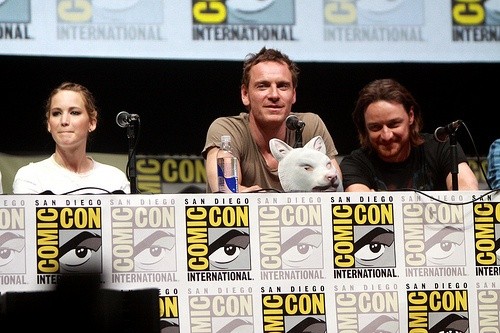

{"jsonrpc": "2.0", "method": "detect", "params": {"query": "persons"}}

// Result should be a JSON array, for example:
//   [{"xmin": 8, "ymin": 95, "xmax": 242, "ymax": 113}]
[
  {"xmin": 13, "ymin": 82, "xmax": 131, "ymax": 195},
  {"xmin": 338, "ymin": 79, "xmax": 478, "ymax": 192},
  {"xmin": 487, "ymin": 139, "xmax": 500, "ymax": 191},
  {"xmin": 201, "ymin": 45, "xmax": 342, "ymax": 194}
]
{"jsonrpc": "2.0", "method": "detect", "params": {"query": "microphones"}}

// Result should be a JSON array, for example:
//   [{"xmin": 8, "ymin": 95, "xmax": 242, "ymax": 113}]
[
  {"xmin": 115, "ymin": 111, "xmax": 139, "ymax": 129},
  {"xmin": 433, "ymin": 119, "xmax": 461, "ymax": 143},
  {"xmin": 284, "ymin": 114, "xmax": 304, "ymax": 130}
]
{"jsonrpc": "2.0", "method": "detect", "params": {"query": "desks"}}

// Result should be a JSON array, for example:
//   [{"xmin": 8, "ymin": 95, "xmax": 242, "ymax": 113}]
[{"xmin": 0, "ymin": 190, "xmax": 500, "ymax": 333}]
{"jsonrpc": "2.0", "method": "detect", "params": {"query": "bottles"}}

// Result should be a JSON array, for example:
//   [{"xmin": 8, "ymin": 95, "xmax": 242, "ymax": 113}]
[{"xmin": 217, "ymin": 135, "xmax": 238, "ymax": 193}]
